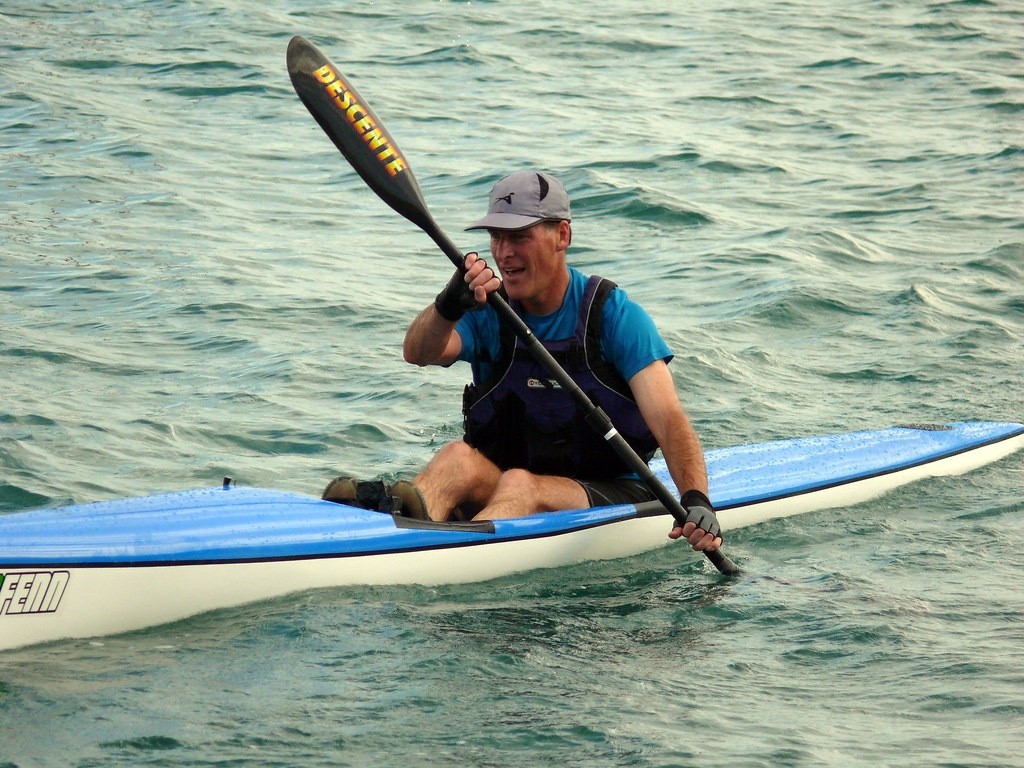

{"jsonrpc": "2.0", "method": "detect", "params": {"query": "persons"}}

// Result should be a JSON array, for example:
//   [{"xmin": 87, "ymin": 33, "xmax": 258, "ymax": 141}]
[{"xmin": 322, "ymin": 171, "xmax": 723, "ymax": 550}]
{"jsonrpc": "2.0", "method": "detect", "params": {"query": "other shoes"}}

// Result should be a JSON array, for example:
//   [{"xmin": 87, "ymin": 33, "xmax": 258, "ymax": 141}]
[
  {"xmin": 387, "ymin": 481, "xmax": 429, "ymax": 522},
  {"xmin": 323, "ymin": 477, "xmax": 358, "ymax": 505}
]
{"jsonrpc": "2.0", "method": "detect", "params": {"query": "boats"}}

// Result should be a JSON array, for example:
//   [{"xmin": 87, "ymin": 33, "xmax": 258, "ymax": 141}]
[{"xmin": 0, "ymin": 420, "xmax": 1024, "ymax": 647}]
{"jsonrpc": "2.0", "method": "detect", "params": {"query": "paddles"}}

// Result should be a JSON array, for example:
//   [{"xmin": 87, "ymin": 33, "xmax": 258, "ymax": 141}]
[{"xmin": 285, "ymin": 28, "xmax": 742, "ymax": 582}]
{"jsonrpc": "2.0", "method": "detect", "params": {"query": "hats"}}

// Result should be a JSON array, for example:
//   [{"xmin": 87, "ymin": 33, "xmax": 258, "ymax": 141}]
[{"xmin": 464, "ymin": 171, "xmax": 572, "ymax": 231}]
